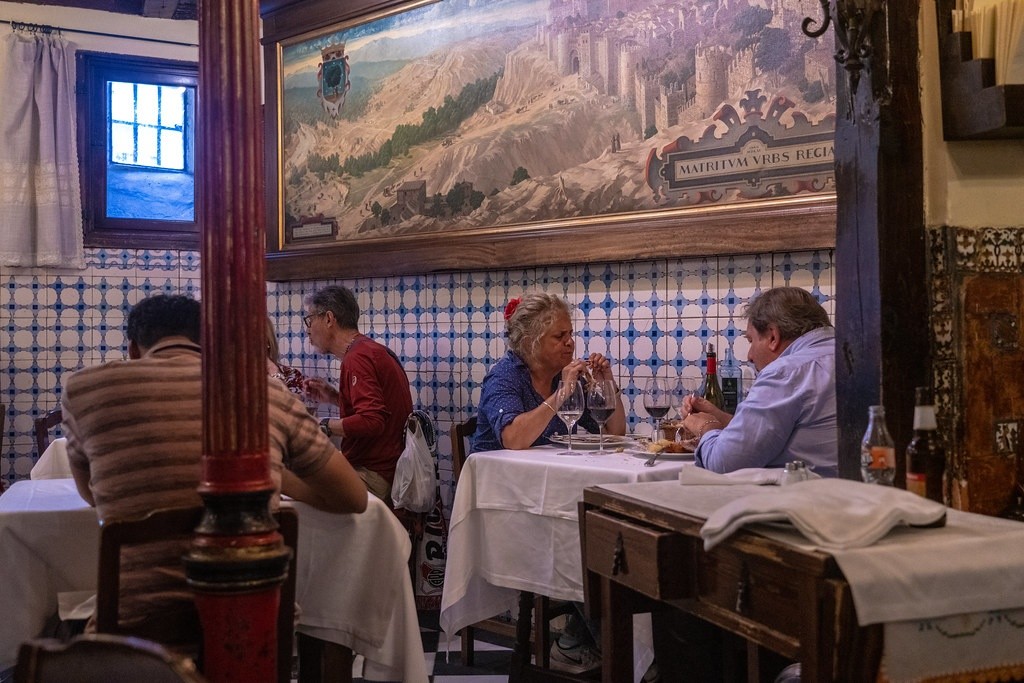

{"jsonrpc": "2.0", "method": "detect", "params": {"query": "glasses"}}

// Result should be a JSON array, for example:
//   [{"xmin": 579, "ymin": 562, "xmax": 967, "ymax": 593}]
[{"xmin": 303, "ymin": 314, "xmax": 315, "ymax": 328}]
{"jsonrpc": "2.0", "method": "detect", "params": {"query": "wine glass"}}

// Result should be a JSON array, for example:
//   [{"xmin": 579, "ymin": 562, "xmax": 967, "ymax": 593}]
[
  {"xmin": 643, "ymin": 377, "xmax": 670, "ymax": 442},
  {"xmin": 587, "ymin": 379, "xmax": 616, "ymax": 456},
  {"xmin": 555, "ymin": 381, "xmax": 585, "ymax": 456}
]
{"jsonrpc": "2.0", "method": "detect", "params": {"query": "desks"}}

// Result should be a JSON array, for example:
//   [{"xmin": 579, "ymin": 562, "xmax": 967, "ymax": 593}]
[
  {"xmin": 30, "ymin": 438, "xmax": 75, "ymax": 480},
  {"xmin": 580, "ymin": 475, "xmax": 1024, "ymax": 683}
]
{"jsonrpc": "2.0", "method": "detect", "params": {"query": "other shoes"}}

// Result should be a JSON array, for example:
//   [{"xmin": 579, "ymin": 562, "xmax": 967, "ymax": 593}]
[{"xmin": 775, "ymin": 663, "xmax": 801, "ymax": 683}]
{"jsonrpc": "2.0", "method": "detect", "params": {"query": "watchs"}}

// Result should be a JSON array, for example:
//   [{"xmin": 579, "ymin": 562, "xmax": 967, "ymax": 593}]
[{"xmin": 318, "ymin": 417, "xmax": 333, "ymax": 438}]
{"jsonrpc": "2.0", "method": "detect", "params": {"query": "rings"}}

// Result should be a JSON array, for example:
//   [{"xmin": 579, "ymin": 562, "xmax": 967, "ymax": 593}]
[{"xmin": 571, "ymin": 361, "xmax": 574, "ymax": 364}]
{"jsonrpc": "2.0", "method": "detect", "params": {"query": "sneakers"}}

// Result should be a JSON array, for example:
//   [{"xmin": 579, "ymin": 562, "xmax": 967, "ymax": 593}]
[{"xmin": 549, "ymin": 638, "xmax": 602, "ymax": 676}]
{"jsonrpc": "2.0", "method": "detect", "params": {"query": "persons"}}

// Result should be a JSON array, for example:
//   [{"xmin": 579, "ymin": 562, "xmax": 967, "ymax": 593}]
[
  {"xmin": 62, "ymin": 293, "xmax": 368, "ymax": 647},
  {"xmin": 475, "ymin": 293, "xmax": 626, "ymax": 674},
  {"xmin": 679, "ymin": 287, "xmax": 838, "ymax": 488},
  {"xmin": 265, "ymin": 286, "xmax": 413, "ymax": 506}
]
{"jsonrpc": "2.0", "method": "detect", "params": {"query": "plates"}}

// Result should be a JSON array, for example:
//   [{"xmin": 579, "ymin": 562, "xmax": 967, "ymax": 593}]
[
  {"xmin": 624, "ymin": 446, "xmax": 695, "ymax": 460},
  {"xmin": 548, "ymin": 434, "xmax": 632, "ymax": 448}
]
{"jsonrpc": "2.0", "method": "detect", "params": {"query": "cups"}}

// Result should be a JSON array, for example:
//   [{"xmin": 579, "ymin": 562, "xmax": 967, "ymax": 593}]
[{"xmin": 670, "ymin": 375, "xmax": 698, "ymax": 421}]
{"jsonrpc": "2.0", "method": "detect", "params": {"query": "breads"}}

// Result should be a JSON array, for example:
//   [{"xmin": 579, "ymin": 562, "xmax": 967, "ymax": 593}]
[{"xmin": 647, "ymin": 439, "xmax": 689, "ymax": 452}]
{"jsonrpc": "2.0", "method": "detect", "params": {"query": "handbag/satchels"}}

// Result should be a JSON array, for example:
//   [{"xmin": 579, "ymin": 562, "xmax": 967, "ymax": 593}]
[{"xmin": 391, "ymin": 419, "xmax": 436, "ymax": 515}]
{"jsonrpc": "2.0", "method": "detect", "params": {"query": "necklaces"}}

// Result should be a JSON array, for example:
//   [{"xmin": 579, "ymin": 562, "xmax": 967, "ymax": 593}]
[{"xmin": 345, "ymin": 333, "xmax": 361, "ymax": 354}]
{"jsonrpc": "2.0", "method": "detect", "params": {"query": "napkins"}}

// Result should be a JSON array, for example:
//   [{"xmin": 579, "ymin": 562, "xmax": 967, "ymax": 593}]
[
  {"xmin": 699, "ymin": 481, "xmax": 949, "ymax": 550},
  {"xmin": 680, "ymin": 462, "xmax": 823, "ymax": 486}
]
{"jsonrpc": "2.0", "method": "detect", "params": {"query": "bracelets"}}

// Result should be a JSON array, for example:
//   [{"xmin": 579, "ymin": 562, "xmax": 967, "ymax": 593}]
[
  {"xmin": 542, "ymin": 402, "xmax": 557, "ymax": 415},
  {"xmin": 698, "ymin": 420, "xmax": 723, "ymax": 441}
]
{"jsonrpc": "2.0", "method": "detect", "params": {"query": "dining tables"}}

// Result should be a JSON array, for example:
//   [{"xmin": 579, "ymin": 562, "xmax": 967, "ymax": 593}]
[
  {"xmin": 445, "ymin": 442, "xmax": 694, "ymax": 683},
  {"xmin": 0, "ymin": 478, "xmax": 430, "ymax": 683}
]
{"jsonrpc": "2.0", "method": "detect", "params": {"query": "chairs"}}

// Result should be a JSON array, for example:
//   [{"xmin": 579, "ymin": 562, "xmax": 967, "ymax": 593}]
[
  {"xmin": 381, "ymin": 409, "xmax": 433, "ymax": 603},
  {"xmin": 32, "ymin": 408, "xmax": 72, "ymax": 458},
  {"xmin": 451, "ymin": 417, "xmax": 567, "ymax": 666},
  {"xmin": 21, "ymin": 632, "xmax": 202, "ymax": 683},
  {"xmin": 59, "ymin": 503, "xmax": 297, "ymax": 681}
]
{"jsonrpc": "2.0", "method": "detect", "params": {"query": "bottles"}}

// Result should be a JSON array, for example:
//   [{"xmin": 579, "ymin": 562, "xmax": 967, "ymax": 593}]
[
  {"xmin": 905, "ymin": 386, "xmax": 947, "ymax": 528},
  {"xmin": 860, "ymin": 406, "xmax": 896, "ymax": 488},
  {"xmin": 781, "ymin": 463, "xmax": 802, "ymax": 486},
  {"xmin": 701, "ymin": 343, "xmax": 725, "ymax": 411},
  {"xmin": 793, "ymin": 460, "xmax": 808, "ymax": 482}
]
